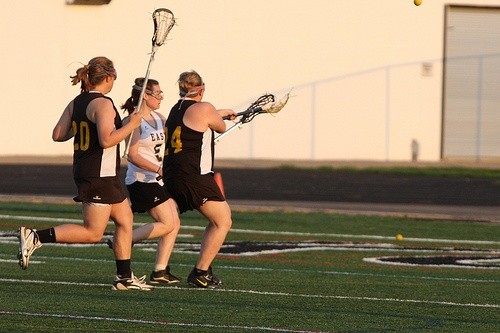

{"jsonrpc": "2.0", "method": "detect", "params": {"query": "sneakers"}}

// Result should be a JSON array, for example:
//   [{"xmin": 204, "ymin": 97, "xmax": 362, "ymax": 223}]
[
  {"xmin": 150, "ymin": 266, "xmax": 182, "ymax": 285},
  {"xmin": 204, "ymin": 265, "xmax": 224, "ymax": 287},
  {"xmin": 186, "ymin": 265, "xmax": 222, "ymax": 290},
  {"xmin": 16, "ymin": 226, "xmax": 43, "ymax": 270},
  {"xmin": 111, "ymin": 271, "xmax": 156, "ymax": 292},
  {"xmin": 107, "ymin": 237, "xmax": 133, "ymax": 261}
]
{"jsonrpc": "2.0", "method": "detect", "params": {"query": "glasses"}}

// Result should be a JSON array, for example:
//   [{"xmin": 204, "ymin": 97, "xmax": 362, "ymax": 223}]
[
  {"xmin": 132, "ymin": 85, "xmax": 164, "ymax": 100},
  {"xmin": 191, "ymin": 83, "xmax": 205, "ymax": 91},
  {"xmin": 106, "ymin": 71, "xmax": 117, "ymax": 81}
]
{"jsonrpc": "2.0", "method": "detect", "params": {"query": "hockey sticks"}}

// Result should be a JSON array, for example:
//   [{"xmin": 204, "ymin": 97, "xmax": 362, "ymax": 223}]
[
  {"xmin": 122, "ymin": 8, "xmax": 177, "ymax": 159},
  {"xmin": 212, "ymin": 91, "xmax": 290, "ymax": 121},
  {"xmin": 213, "ymin": 95, "xmax": 276, "ymax": 143}
]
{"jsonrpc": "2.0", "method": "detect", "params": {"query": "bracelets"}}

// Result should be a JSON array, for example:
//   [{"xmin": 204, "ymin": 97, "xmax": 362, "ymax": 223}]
[{"xmin": 155, "ymin": 165, "xmax": 161, "ymax": 173}]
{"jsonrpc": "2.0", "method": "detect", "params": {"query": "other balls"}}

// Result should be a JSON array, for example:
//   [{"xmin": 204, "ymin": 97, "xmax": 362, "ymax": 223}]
[
  {"xmin": 396, "ymin": 233, "xmax": 404, "ymax": 241},
  {"xmin": 414, "ymin": 0, "xmax": 422, "ymax": 6}
]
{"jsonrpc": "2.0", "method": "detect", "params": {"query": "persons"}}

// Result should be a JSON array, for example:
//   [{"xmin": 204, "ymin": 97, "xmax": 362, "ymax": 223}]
[
  {"xmin": 108, "ymin": 78, "xmax": 182, "ymax": 284},
  {"xmin": 162, "ymin": 71, "xmax": 238, "ymax": 290},
  {"xmin": 18, "ymin": 57, "xmax": 155, "ymax": 291}
]
